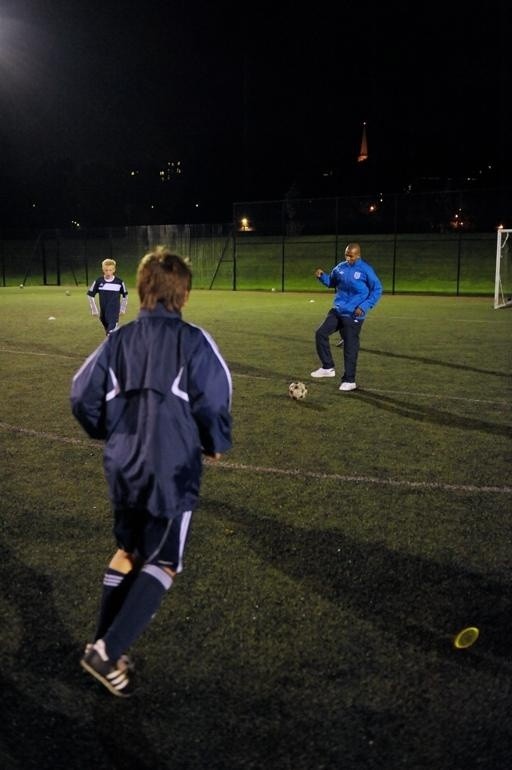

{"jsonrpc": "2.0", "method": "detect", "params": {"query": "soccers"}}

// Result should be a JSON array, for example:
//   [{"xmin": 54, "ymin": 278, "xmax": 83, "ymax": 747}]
[{"xmin": 289, "ymin": 382, "xmax": 308, "ymax": 400}]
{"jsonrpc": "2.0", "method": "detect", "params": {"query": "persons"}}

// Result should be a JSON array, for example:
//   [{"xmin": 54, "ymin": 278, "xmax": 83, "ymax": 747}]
[
  {"xmin": 85, "ymin": 257, "xmax": 128, "ymax": 335},
  {"xmin": 310, "ymin": 242, "xmax": 383, "ymax": 392},
  {"xmin": 68, "ymin": 252, "xmax": 234, "ymax": 700}
]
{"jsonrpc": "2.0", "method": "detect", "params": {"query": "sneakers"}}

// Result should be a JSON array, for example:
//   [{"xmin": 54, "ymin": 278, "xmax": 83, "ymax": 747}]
[
  {"xmin": 80, "ymin": 639, "xmax": 143, "ymax": 699},
  {"xmin": 310, "ymin": 367, "xmax": 336, "ymax": 378},
  {"xmin": 339, "ymin": 382, "xmax": 357, "ymax": 391}
]
